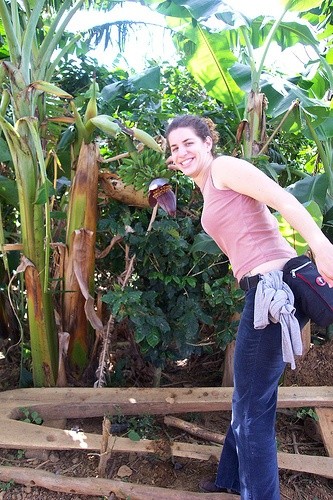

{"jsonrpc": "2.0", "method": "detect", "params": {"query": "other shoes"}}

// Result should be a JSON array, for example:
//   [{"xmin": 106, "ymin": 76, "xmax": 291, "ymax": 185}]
[{"xmin": 199, "ymin": 478, "xmax": 241, "ymax": 495}]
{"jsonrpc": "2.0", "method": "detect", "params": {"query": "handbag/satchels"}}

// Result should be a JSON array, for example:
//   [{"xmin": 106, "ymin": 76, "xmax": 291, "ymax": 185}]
[{"xmin": 279, "ymin": 255, "xmax": 333, "ymax": 327}]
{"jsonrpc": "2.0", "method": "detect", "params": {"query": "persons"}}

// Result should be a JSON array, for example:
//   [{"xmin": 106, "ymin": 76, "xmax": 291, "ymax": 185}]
[{"xmin": 164, "ymin": 115, "xmax": 332, "ymax": 500}]
